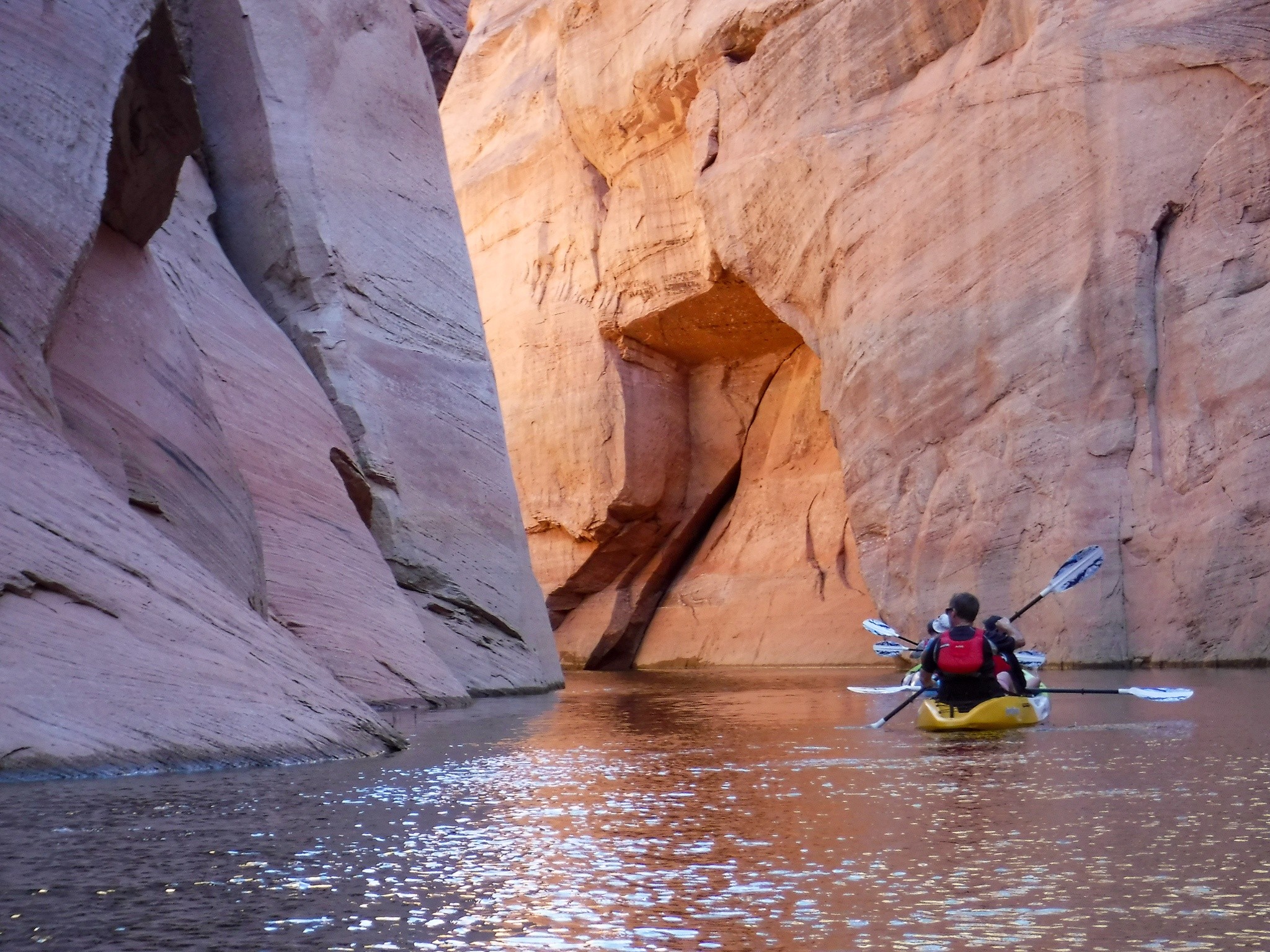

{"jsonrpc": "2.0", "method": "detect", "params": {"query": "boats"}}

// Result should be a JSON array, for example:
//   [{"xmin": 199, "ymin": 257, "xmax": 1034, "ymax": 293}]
[{"xmin": 915, "ymin": 669, "xmax": 1052, "ymax": 734}]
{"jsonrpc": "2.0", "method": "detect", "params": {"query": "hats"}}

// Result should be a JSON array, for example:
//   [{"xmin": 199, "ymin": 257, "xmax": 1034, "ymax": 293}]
[
  {"xmin": 933, "ymin": 614, "xmax": 950, "ymax": 633},
  {"xmin": 982, "ymin": 615, "xmax": 1007, "ymax": 635}
]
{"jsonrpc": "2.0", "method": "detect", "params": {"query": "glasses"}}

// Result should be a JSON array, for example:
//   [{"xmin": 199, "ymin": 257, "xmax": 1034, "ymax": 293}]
[{"xmin": 945, "ymin": 607, "xmax": 956, "ymax": 615}]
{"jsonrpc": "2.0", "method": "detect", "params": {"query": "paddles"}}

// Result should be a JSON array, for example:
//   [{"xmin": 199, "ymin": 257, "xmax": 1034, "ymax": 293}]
[
  {"xmin": 846, "ymin": 685, "xmax": 1195, "ymax": 703},
  {"xmin": 862, "ymin": 544, "xmax": 1106, "ymax": 729},
  {"xmin": 863, "ymin": 619, "xmax": 918, "ymax": 646},
  {"xmin": 873, "ymin": 642, "xmax": 1047, "ymax": 669}
]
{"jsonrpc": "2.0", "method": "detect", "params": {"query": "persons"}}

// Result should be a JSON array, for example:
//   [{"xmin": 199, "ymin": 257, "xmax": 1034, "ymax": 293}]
[
  {"xmin": 920, "ymin": 592, "xmax": 1026, "ymax": 711},
  {"xmin": 980, "ymin": 615, "xmax": 1041, "ymax": 696},
  {"xmin": 910, "ymin": 613, "xmax": 950, "ymax": 690}
]
